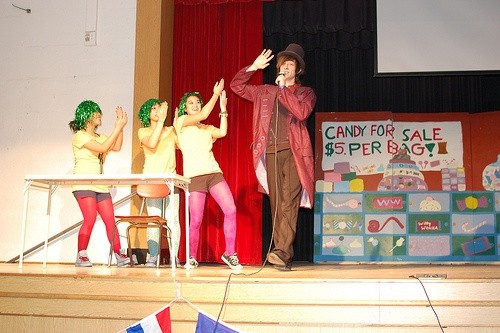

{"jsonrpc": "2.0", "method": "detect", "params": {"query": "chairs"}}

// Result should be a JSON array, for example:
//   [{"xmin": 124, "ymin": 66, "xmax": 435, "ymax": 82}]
[{"xmin": 108, "ymin": 183, "xmax": 179, "ymax": 270}]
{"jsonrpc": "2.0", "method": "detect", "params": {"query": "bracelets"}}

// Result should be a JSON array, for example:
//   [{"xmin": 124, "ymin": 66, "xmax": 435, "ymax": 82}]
[
  {"xmin": 219, "ymin": 111, "xmax": 228, "ymax": 118},
  {"xmin": 158, "ymin": 120, "xmax": 164, "ymax": 123}
]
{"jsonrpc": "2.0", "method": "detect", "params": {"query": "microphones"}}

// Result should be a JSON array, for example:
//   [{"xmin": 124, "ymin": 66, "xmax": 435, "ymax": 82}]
[{"xmin": 278, "ymin": 71, "xmax": 287, "ymax": 77}]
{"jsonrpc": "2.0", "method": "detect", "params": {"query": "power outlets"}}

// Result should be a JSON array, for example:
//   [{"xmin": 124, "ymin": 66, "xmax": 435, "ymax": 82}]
[{"xmin": 85, "ymin": 32, "xmax": 97, "ymax": 45}]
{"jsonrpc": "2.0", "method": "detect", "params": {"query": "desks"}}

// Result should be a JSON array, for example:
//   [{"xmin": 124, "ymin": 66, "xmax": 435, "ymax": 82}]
[{"xmin": 17, "ymin": 172, "xmax": 190, "ymax": 271}]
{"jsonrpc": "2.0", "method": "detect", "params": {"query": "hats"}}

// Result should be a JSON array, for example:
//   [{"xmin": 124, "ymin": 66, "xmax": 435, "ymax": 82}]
[{"xmin": 277, "ymin": 43, "xmax": 305, "ymax": 78}]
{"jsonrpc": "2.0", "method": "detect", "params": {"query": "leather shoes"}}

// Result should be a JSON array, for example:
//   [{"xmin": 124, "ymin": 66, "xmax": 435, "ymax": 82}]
[{"xmin": 267, "ymin": 249, "xmax": 291, "ymax": 271}]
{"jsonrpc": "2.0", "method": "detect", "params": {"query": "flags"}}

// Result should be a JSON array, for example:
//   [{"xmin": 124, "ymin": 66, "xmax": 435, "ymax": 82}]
[
  {"xmin": 195, "ymin": 311, "xmax": 239, "ymax": 333},
  {"xmin": 127, "ymin": 306, "xmax": 171, "ymax": 333}
]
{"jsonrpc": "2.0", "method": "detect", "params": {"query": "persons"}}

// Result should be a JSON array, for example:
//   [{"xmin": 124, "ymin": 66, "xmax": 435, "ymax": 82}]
[
  {"xmin": 173, "ymin": 77, "xmax": 244, "ymax": 270},
  {"xmin": 68, "ymin": 100, "xmax": 134, "ymax": 267},
  {"xmin": 229, "ymin": 43, "xmax": 317, "ymax": 271},
  {"xmin": 138, "ymin": 98, "xmax": 182, "ymax": 267}
]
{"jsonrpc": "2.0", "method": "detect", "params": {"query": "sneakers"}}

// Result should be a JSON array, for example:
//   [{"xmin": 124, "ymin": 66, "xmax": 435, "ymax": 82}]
[
  {"xmin": 184, "ymin": 256, "xmax": 199, "ymax": 268},
  {"xmin": 221, "ymin": 251, "xmax": 243, "ymax": 269},
  {"xmin": 145, "ymin": 255, "xmax": 157, "ymax": 268},
  {"xmin": 75, "ymin": 250, "xmax": 92, "ymax": 267},
  {"xmin": 114, "ymin": 249, "xmax": 135, "ymax": 267},
  {"xmin": 170, "ymin": 257, "xmax": 181, "ymax": 267}
]
